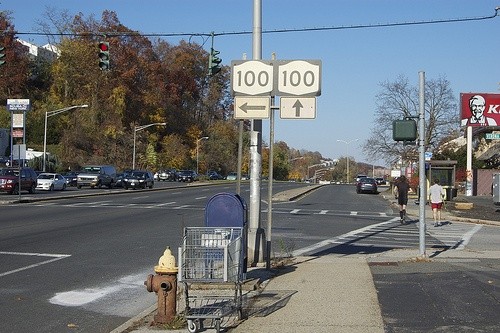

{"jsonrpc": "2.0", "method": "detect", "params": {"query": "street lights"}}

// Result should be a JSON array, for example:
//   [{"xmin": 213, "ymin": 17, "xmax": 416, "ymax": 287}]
[
  {"xmin": 132, "ymin": 123, "xmax": 167, "ymax": 172},
  {"xmin": 196, "ymin": 136, "xmax": 209, "ymax": 177},
  {"xmin": 43, "ymin": 105, "xmax": 89, "ymax": 173},
  {"xmin": 336, "ymin": 138, "xmax": 359, "ymax": 183}
]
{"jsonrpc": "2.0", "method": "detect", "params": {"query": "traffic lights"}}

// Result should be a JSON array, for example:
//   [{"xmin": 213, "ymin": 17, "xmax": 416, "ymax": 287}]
[
  {"xmin": 208, "ymin": 58, "xmax": 222, "ymax": 78},
  {"xmin": 98, "ymin": 41, "xmax": 111, "ymax": 71},
  {"xmin": 210, "ymin": 49, "xmax": 220, "ymax": 70}
]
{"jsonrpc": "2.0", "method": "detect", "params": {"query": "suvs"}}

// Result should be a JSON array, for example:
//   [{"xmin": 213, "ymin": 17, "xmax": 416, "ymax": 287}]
[
  {"xmin": 0, "ymin": 168, "xmax": 36, "ymax": 196},
  {"xmin": 78, "ymin": 165, "xmax": 117, "ymax": 190}
]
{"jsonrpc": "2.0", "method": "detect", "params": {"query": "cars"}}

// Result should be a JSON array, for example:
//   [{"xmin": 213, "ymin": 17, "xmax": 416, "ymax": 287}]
[
  {"xmin": 357, "ymin": 178, "xmax": 377, "ymax": 194},
  {"xmin": 354, "ymin": 175, "xmax": 367, "ymax": 184},
  {"xmin": 63, "ymin": 172, "xmax": 79, "ymax": 187},
  {"xmin": 34, "ymin": 173, "xmax": 67, "ymax": 191},
  {"xmin": 207, "ymin": 170, "xmax": 252, "ymax": 181},
  {"xmin": 376, "ymin": 178, "xmax": 386, "ymax": 185},
  {"xmin": 114, "ymin": 169, "xmax": 200, "ymax": 188}
]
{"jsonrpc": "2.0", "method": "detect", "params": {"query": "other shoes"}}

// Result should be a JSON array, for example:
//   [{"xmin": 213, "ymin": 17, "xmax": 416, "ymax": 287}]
[
  {"xmin": 403, "ymin": 221, "xmax": 406, "ymax": 224},
  {"xmin": 434, "ymin": 222, "xmax": 438, "ymax": 227},
  {"xmin": 400, "ymin": 219, "xmax": 403, "ymax": 223},
  {"xmin": 438, "ymin": 221, "xmax": 442, "ymax": 226}
]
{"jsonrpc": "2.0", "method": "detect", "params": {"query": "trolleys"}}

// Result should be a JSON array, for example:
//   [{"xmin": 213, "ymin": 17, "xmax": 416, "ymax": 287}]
[{"xmin": 178, "ymin": 227, "xmax": 249, "ymax": 333}]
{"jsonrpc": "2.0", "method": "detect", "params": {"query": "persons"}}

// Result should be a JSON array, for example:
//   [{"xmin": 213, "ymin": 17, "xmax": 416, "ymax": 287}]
[
  {"xmin": 428, "ymin": 179, "xmax": 445, "ymax": 227},
  {"xmin": 394, "ymin": 175, "xmax": 412, "ymax": 224}
]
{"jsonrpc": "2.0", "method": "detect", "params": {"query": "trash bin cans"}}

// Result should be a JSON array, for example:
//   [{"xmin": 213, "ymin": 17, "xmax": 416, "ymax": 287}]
[
  {"xmin": 447, "ymin": 188, "xmax": 457, "ymax": 201},
  {"xmin": 203, "ymin": 191, "xmax": 248, "ymax": 282}
]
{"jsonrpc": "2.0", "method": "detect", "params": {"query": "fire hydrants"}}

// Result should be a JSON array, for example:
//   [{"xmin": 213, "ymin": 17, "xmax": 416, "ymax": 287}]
[{"xmin": 142, "ymin": 246, "xmax": 182, "ymax": 325}]
